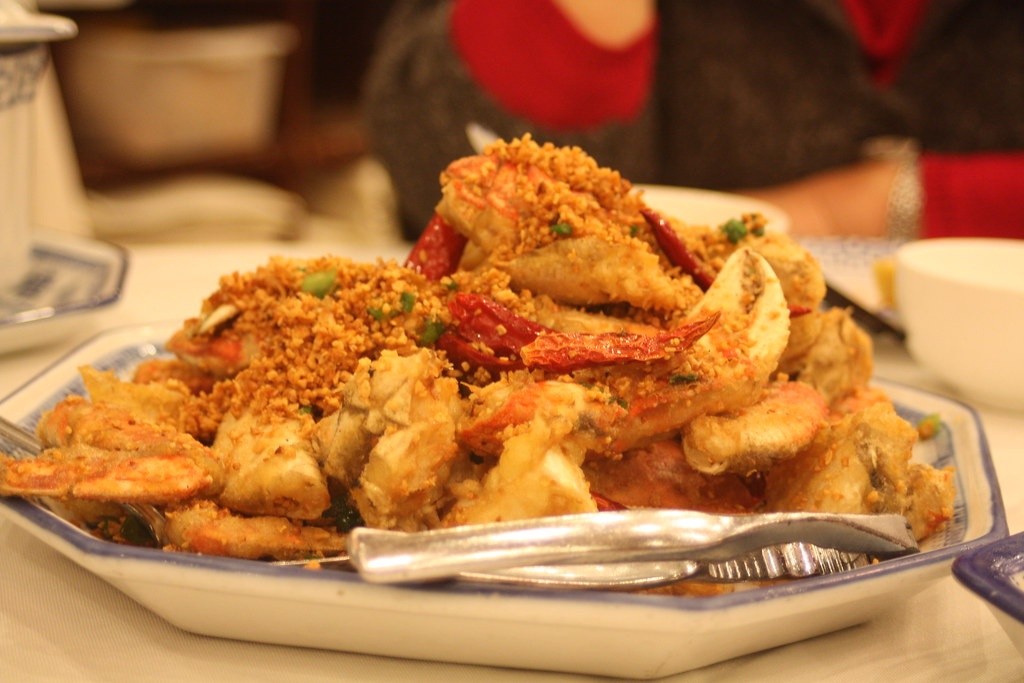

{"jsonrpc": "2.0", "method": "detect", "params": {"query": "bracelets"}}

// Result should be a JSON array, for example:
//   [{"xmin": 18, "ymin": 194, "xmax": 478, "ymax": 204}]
[{"xmin": 865, "ymin": 138, "xmax": 922, "ymax": 242}]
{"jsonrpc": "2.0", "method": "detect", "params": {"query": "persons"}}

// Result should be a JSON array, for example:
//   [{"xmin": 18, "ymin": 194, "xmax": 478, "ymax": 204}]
[{"xmin": 363, "ymin": 0, "xmax": 1024, "ymax": 243}]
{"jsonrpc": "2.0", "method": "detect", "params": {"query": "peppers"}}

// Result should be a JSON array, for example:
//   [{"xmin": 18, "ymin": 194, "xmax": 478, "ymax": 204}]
[{"xmin": 400, "ymin": 200, "xmax": 816, "ymax": 373}]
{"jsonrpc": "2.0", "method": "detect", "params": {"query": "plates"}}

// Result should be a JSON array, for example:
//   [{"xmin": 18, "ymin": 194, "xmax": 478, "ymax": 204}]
[
  {"xmin": 791, "ymin": 231, "xmax": 900, "ymax": 337},
  {"xmin": 1, "ymin": 323, "xmax": 1012, "ymax": 682},
  {"xmin": 0, "ymin": 226, "xmax": 130, "ymax": 360},
  {"xmin": 950, "ymin": 529, "xmax": 1024, "ymax": 668}
]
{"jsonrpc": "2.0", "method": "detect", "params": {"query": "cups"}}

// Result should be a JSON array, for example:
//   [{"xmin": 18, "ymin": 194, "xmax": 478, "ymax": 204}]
[{"xmin": 0, "ymin": 9, "xmax": 79, "ymax": 291}]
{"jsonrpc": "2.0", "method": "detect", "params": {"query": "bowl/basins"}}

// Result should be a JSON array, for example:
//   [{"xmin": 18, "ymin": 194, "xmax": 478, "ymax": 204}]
[
  {"xmin": 893, "ymin": 233, "xmax": 1024, "ymax": 414},
  {"xmin": 618, "ymin": 181, "xmax": 794, "ymax": 243}
]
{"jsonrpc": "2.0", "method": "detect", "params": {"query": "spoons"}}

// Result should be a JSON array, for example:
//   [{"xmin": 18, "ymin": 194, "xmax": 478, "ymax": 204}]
[{"xmin": 1, "ymin": 414, "xmax": 349, "ymax": 568}]
